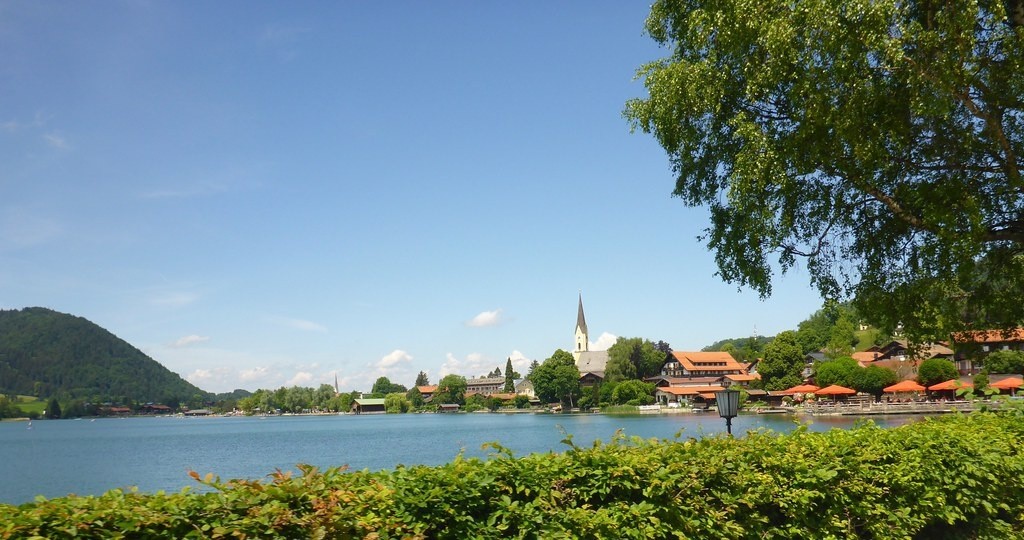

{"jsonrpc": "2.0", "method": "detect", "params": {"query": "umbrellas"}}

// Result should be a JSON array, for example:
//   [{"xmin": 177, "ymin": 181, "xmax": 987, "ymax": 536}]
[
  {"xmin": 815, "ymin": 385, "xmax": 855, "ymax": 403},
  {"xmin": 883, "ymin": 380, "xmax": 925, "ymax": 395},
  {"xmin": 928, "ymin": 380, "xmax": 974, "ymax": 400},
  {"xmin": 783, "ymin": 384, "xmax": 819, "ymax": 407},
  {"xmin": 988, "ymin": 378, "xmax": 1024, "ymax": 397}
]
